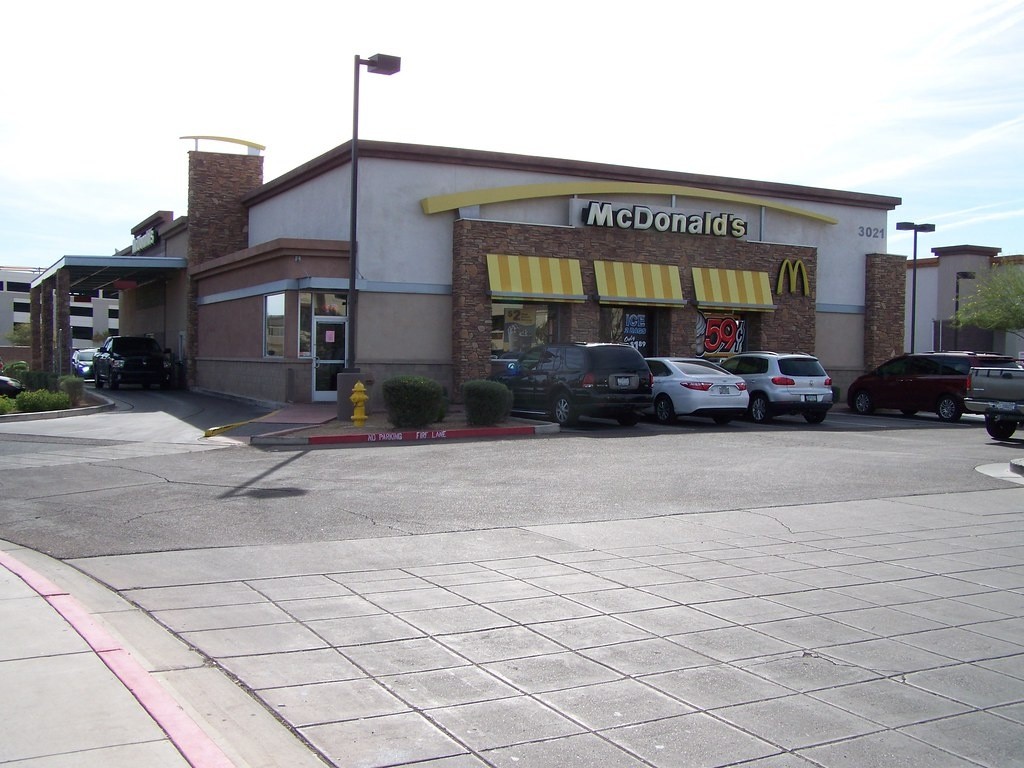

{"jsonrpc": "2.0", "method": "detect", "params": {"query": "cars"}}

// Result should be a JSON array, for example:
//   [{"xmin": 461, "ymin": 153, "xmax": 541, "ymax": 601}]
[
  {"xmin": 72, "ymin": 348, "xmax": 97, "ymax": 380},
  {"xmin": 644, "ymin": 357, "xmax": 749, "ymax": 426},
  {"xmin": 0, "ymin": 376, "xmax": 26, "ymax": 400}
]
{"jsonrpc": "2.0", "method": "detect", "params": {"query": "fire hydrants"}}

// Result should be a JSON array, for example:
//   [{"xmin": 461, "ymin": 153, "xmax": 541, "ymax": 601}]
[{"xmin": 349, "ymin": 380, "xmax": 369, "ymax": 428}]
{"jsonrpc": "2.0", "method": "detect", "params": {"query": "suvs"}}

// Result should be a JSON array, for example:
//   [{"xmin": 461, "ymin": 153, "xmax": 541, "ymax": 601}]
[
  {"xmin": 485, "ymin": 341, "xmax": 655, "ymax": 428},
  {"xmin": 846, "ymin": 349, "xmax": 1024, "ymax": 423},
  {"xmin": 715, "ymin": 350, "xmax": 835, "ymax": 425},
  {"xmin": 93, "ymin": 334, "xmax": 176, "ymax": 392}
]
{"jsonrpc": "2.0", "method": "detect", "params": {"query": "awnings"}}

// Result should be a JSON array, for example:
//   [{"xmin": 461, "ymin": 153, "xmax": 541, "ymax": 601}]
[
  {"xmin": 593, "ymin": 259, "xmax": 689, "ymax": 309},
  {"xmin": 692, "ymin": 266, "xmax": 777, "ymax": 311},
  {"xmin": 485, "ymin": 251, "xmax": 588, "ymax": 303}
]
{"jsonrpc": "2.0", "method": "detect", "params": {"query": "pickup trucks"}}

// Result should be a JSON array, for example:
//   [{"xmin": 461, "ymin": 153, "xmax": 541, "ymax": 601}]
[{"xmin": 963, "ymin": 366, "xmax": 1024, "ymax": 441}]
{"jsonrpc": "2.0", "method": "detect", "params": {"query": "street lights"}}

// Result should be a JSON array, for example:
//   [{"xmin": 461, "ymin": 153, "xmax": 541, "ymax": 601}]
[
  {"xmin": 955, "ymin": 271, "xmax": 976, "ymax": 351},
  {"xmin": 342, "ymin": 53, "xmax": 403, "ymax": 371},
  {"xmin": 896, "ymin": 222, "xmax": 936, "ymax": 354}
]
